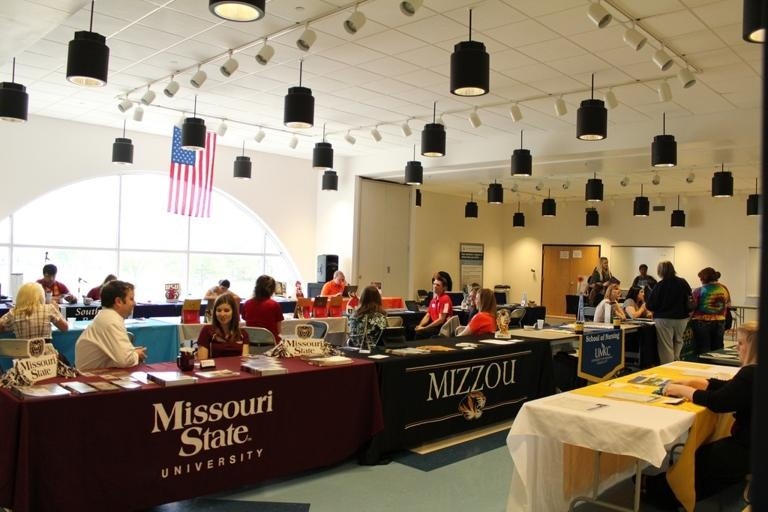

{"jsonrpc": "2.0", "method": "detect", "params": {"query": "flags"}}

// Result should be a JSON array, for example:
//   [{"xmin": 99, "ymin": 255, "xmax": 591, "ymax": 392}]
[{"xmin": 167, "ymin": 126, "xmax": 218, "ymax": 218}]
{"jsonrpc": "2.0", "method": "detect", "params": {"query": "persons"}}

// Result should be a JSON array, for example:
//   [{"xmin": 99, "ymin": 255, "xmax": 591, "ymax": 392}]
[
  {"xmin": 584, "ymin": 257, "xmax": 733, "ymax": 363},
  {"xmin": 197, "ymin": 276, "xmax": 284, "ymax": 360},
  {"xmin": 0, "ymin": 265, "xmax": 147, "ymax": 371},
  {"xmin": 321, "ymin": 270, "xmax": 389, "ymax": 349},
  {"xmin": 662, "ymin": 319, "xmax": 767, "ymax": 512},
  {"xmin": 415, "ymin": 273, "xmax": 498, "ymax": 336}
]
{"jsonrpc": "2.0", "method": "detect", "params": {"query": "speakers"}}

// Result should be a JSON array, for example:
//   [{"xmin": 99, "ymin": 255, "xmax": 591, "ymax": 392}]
[{"xmin": 316, "ymin": 254, "xmax": 338, "ymax": 282}]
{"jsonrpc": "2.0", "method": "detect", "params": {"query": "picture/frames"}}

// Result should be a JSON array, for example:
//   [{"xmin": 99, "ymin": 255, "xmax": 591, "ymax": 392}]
[{"xmin": 460, "ymin": 243, "xmax": 484, "ymax": 291}]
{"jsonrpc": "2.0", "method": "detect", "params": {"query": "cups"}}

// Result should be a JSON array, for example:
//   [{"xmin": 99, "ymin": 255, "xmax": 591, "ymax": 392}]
[
  {"xmin": 537, "ymin": 319, "xmax": 544, "ymax": 329},
  {"xmin": 177, "ymin": 348, "xmax": 195, "ymax": 372},
  {"xmin": 83, "ymin": 297, "xmax": 94, "ymax": 305}
]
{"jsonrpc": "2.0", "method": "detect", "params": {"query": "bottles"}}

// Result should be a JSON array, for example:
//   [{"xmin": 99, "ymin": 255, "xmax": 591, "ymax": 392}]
[
  {"xmin": 521, "ymin": 293, "xmax": 527, "ymax": 306},
  {"xmin": 575, "ymin": 321, "xmax": 584, "ymax": 334},
  {"xmin": 613, "ymin": 317, "xmax": 621, "ymax": 329},
  {"xmin": 45, "ymin": 290, "xmax": 52, "ymax": 303},
  {"xmin": 605, "ymin": 303, "xmax": 611, "ymax": 323},
  {"xmin": 204, "ymin": 310, "xmax": 211, "ymax": 324}
]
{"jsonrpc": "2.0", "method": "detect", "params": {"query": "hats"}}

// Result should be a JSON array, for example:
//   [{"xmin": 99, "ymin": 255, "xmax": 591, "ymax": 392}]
[{"xmin": 432, "ymin": 271, "xmax": 452, "ymax": 292}]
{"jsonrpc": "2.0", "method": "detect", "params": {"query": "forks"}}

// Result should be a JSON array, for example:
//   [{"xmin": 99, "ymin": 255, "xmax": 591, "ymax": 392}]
[{"xmin": 587, "ymin": 403, "xmax": 608, "ymax": 411}]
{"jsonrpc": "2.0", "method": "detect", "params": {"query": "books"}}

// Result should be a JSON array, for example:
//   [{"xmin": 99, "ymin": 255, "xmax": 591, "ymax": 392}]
[{"xmin": 147, "ymin": 371, "xmax": 199, "ymax": 387}]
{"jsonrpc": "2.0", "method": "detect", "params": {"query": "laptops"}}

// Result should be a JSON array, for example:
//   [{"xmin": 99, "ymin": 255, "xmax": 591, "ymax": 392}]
[
  {"xmin": 342, "ymin": 286, "xmax": 358, "ymax": 297},
  {"xmin": 405, "ymin": 300, "xmax": 425, "ymax": 313}
]
